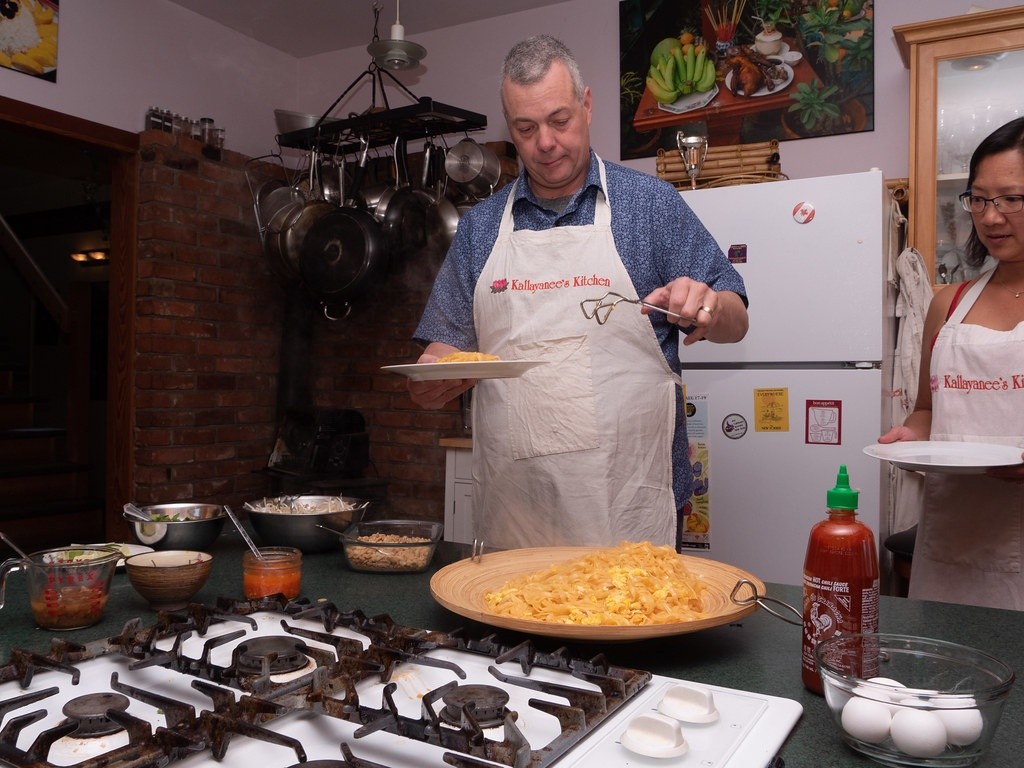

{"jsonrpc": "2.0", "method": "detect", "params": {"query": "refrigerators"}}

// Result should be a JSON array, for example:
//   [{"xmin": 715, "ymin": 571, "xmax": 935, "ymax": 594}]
[{"xmin": 677, "ymin": 170, "xmax": 905, "ymax": 595}]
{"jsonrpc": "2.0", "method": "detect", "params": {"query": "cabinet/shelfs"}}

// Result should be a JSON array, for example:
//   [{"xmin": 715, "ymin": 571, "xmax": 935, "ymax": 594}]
[
  {"xmin": 445, "ymin": 448, "xmax": 475, "ymax": 545},
  {"xmin": 890, "ymin": 5, "xmax": 1024, "ymax": 306}
]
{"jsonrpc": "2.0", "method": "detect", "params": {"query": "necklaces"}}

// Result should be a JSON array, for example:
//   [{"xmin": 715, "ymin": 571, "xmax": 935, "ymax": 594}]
[{"xmin": 994, "ymin": 274, "xmax": 1023, "ymax": 298}]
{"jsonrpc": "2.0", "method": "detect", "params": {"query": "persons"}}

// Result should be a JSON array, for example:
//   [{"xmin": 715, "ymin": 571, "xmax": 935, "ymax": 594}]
[
  {"xmin": 405, "ymin": 33, "xmax": 749, "ymax": 554},
  {"xmin": 875, "ymin": 115, "xmax": 1024, "ymax": 613}
]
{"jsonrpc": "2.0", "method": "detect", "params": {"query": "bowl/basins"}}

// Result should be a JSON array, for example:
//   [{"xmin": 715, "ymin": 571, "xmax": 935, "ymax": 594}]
[
  {"xmin": 813, "ymin": 633, "xmax": 1015, "ymax": 768},
  {"xmin": 130, "ymin": 502, "xmax": 227, "ymax": 549},
  {"xmin": 125, "ymin": 551, "xmax": 212, "ymax": 611},
  {"xmin": 782, "ymin": 50, "xmax": 803, "ymax": 67},
  {"xmin": 242, "ymin": 495, "xmax": 371, "ymax": 552},
  {"xmin": 341, "ymin": 520, "xmax": 445, "ymax": 571}
]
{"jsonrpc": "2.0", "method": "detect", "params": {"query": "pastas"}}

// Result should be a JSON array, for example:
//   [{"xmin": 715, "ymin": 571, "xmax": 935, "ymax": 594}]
[
  {"xmin": 437, "ymin": 351, "xmax": 502, "ymax": 361},
  {"xmin": 486, "ymin": 541, "xmax": 707, "ymax": 626}
]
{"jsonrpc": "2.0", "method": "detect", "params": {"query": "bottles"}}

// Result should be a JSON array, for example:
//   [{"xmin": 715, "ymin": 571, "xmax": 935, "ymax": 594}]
[
  {"xmin": 146, "ymin": 105, "xmax": 225, "ymax": 161},
  {"xmin": 242, "ymin": 547, "xmax": 302, "ymax": 601},
  {"xmin": 800, "ymin": 464, "xmax": 880, "ymax": 695}
]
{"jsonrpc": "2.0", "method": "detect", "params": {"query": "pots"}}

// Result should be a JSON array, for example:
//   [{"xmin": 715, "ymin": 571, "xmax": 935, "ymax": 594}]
[{"xmin": 245, "ymin": 108, "xmax": 502, "ymax": 320}]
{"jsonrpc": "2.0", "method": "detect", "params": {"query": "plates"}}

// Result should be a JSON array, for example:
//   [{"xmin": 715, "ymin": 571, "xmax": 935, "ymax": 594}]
[
  {"xmin": 657, "ymin": 42, "xmax": 803, "ymax": 115},
  {"xmin": 85, "ymin": 543, "xmax": 155, "ymax": 566},
  {"xmin": 380, "ymin": 358, "xmax": 547, "ymax": 379},
  {"xmin": 864, "ymin": 442, "xmax": 1024, "ymax": 472}
]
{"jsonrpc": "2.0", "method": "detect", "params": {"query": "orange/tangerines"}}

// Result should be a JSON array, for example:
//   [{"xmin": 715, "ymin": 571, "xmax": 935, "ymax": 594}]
[{"xmin": 678, "ymin": 32, "xmax": 706, "ymax": 62}]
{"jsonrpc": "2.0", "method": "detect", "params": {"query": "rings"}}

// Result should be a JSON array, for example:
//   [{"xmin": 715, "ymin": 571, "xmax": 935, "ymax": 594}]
[{"xmin": 701, "ymin": 306, "xmax": 714, "ymax": 318}]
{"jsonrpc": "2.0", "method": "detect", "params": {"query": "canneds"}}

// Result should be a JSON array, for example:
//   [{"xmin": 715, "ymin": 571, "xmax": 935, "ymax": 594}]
[{"xmin": 243, "ymin": 547, "xmax": 302, "ymax": 601}]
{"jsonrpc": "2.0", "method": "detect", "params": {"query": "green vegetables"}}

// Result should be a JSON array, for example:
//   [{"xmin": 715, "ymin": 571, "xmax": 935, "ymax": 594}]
[{"xmin": 147, "ymin": 513, "xmax": 194, "ymax": 521}]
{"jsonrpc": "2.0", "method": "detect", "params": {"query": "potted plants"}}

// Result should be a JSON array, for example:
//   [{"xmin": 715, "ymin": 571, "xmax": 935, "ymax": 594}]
[
  {"xmin": 619, "ymin": 0, "xmax": 874, "ymax": 158},
  {"xmin": 780, "ymin": 79, "xmax": 866, "ymax": 139}
]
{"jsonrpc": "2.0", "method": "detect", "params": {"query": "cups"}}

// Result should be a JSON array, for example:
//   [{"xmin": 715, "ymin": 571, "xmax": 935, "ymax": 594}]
[
  {"xmin": 0, "ymin": 548, "xmax": 125, "ymax": 630},
  {"xmin": 755, "ymin": 30, "xmax": 782, "ymax": 54}
]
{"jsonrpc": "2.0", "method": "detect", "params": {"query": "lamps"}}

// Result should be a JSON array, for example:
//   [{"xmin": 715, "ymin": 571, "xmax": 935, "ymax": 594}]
[{"xmin": 365, "ymin": 0, "xmax": 428, "ymax": 72}]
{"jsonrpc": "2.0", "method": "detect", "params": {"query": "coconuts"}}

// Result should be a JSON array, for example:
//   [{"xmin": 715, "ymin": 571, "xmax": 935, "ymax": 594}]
[{"xmin": 650, "ymin": 38, "xmax": 681, "ymax": 66}]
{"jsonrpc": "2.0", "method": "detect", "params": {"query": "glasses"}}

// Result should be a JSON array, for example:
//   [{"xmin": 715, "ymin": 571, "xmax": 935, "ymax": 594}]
[{"xmin": 959, "ymin": 191, "xmax": 1024, "ymax": 214}]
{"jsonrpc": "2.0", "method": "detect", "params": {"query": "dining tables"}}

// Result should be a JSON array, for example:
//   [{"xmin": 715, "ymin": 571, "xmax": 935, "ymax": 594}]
[{"xmin": 632, "ymin": 38, "xmax": 824, "ymax": 146}]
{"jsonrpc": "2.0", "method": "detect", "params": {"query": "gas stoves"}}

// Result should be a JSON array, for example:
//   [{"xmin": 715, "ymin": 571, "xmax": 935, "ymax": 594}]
[{"xmin": 0, "ymin": 592, "xmax": 804, "ymax": 768}]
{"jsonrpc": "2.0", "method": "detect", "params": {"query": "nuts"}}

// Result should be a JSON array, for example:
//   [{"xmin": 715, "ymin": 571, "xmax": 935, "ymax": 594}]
[{"xmin": 347, "ymin": 533, "xmax": 431, "ymax": 571}]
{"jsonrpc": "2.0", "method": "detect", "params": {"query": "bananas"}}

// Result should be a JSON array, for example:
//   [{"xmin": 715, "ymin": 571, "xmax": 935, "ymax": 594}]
[{"xmin": 644, "ymin": 45, "xmax": 716, "ymax": 103}]
